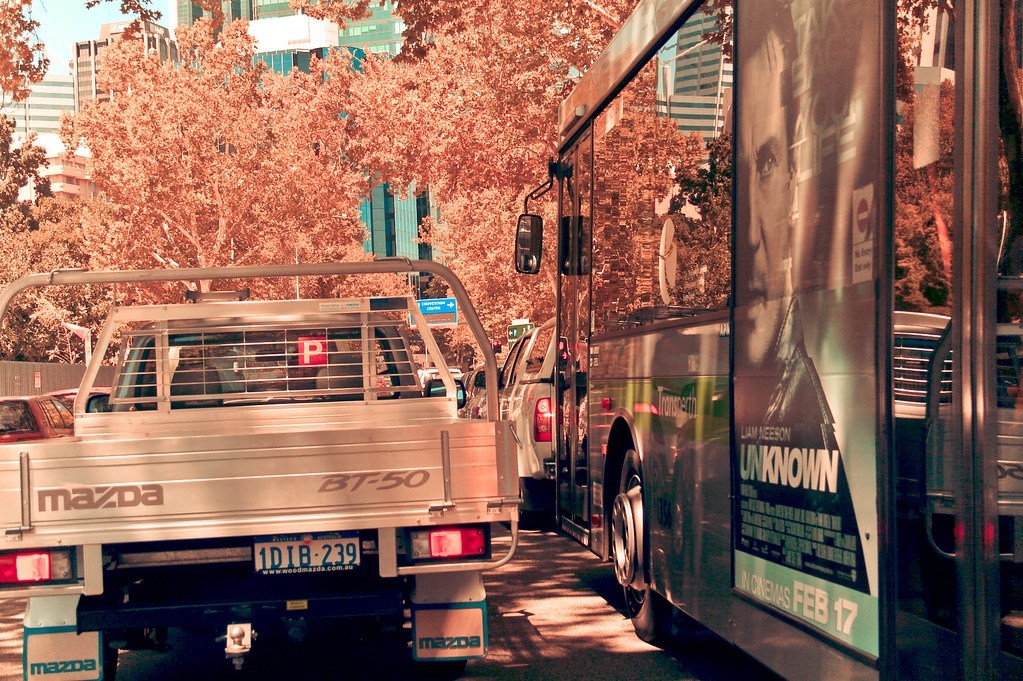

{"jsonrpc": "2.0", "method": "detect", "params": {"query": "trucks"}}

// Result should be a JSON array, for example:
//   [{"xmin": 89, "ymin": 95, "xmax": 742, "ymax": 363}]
[
  {"xmin": 927, "ymin": 279, "xmax": 1022, "ymax": 563},
  {"xmin": 0, "ymin": 257, "xmax": 523, "ymax": 681}
]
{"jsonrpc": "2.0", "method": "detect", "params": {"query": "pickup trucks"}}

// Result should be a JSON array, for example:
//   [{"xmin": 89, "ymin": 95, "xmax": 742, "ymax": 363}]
[{"xmin": 474, "ymin": 315, "xmax": 560, "ymax": 530}]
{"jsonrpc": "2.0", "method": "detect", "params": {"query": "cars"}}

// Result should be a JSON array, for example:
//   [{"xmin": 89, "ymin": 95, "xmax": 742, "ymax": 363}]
[
  {"xmin": 416, "ymin": 368, "xmax": 499, "ymax": 419},
  {"xmin": 0, "ymin": 394, "xmax": 78, "ymax": 447}
]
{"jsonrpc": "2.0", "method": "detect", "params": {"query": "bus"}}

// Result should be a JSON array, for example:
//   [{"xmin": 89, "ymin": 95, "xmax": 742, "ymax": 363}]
[{"xmin": 514, "ymin": 0, "xmax": 1023, "ymax": 681}]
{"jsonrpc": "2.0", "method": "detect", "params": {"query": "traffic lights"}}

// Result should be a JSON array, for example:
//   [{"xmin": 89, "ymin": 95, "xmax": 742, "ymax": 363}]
[
  {"xmin": 559, "ymin": 337, "xmax": 571, "ymax": 364},
  {"xmin": 492, "ymin": 340, "xmax": 502, "ymax": 354}
]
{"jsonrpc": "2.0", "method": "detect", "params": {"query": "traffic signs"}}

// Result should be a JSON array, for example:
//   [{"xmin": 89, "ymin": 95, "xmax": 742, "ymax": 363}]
[{"xmin": 411, "ymin": 298, "xmax": 458, "ymax": 326}]
